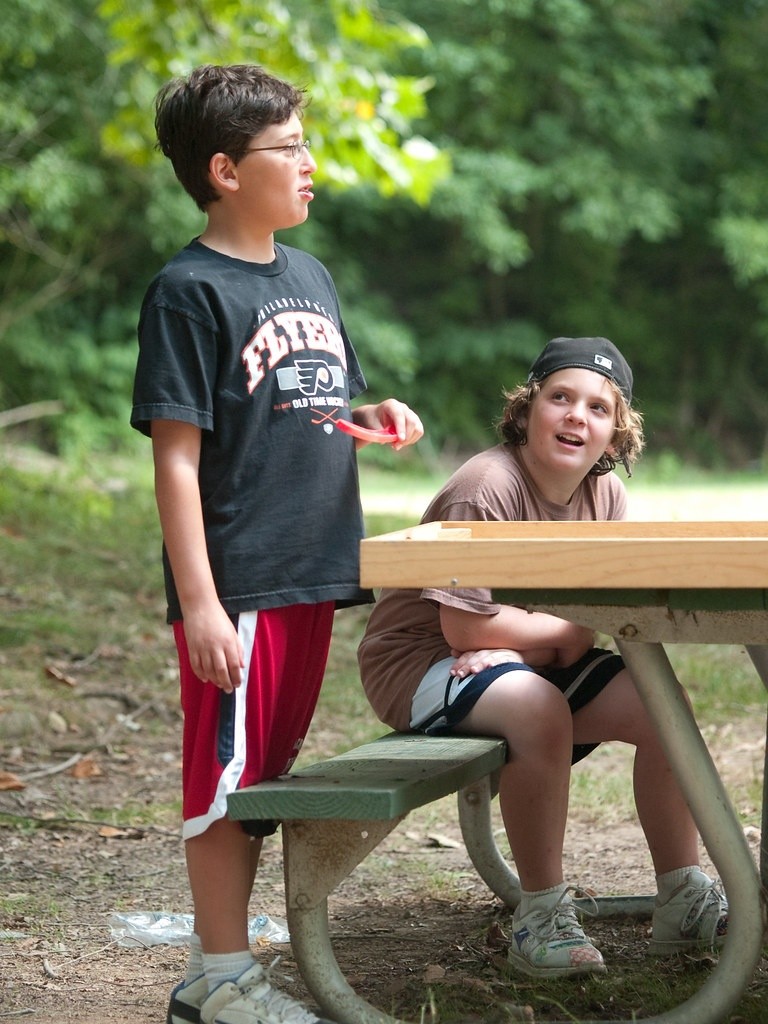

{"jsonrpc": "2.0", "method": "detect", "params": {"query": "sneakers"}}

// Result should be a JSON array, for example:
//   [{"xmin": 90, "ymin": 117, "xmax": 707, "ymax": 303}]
[
  {"xmin": 167, "ymin": 963, "xmax": 336, "ymax": 1023},
  {"xmin": 648, "ymin": 872, "xmax": 729, "ymax": 956},
  {"xmin": 508, "ymin": 893, "xmax": 609, "ymax": 978}
]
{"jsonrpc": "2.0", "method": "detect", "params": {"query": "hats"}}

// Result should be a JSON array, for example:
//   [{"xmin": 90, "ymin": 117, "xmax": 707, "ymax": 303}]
[{"xmin": 529, "ymin": 337, "xmax": 633, "ymax": 404}]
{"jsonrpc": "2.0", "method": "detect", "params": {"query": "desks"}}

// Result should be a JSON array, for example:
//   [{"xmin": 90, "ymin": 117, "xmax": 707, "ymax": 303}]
[{"xmin": 358, "ymin": 518, "xmax": 767, "ymax": 1024}]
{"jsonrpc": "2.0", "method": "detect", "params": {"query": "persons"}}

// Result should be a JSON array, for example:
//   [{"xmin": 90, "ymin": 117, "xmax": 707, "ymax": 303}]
[
  {"xmin": 359, "ymin": 338, "xmax": 731, "ymax": 978},
  {"xmin": 133, "ymin": 64, "xmax": 424, "ymax": 1024}
]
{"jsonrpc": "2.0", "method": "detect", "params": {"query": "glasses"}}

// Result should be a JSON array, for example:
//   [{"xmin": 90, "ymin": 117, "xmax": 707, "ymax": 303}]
[{"xmin": 231, "ymin": 139, "xmax": 311, "ymax": 159}]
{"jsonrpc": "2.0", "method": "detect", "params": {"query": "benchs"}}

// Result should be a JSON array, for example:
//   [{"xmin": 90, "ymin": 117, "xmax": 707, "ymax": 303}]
[{"xmin": 226, "ymin": 726, "xmax": 541, "ymax": 1024}]
